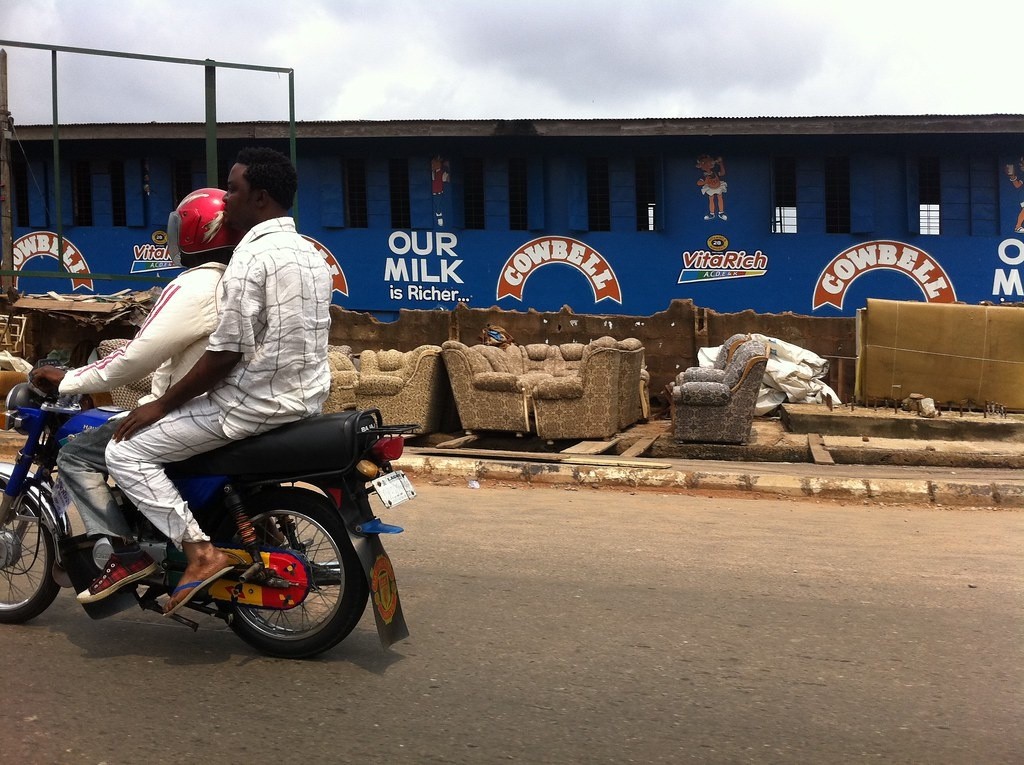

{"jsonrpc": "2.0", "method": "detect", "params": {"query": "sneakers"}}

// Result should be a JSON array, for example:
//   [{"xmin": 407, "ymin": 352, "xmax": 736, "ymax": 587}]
[{"xmin": 77, "ymin": 549, "xmax": 156, "ymax": 603}]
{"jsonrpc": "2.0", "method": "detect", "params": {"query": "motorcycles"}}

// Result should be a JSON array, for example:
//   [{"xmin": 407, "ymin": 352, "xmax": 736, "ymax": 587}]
[{"xmin": 0, "ymin": 359, "xmax": 417, "ymax": 662}]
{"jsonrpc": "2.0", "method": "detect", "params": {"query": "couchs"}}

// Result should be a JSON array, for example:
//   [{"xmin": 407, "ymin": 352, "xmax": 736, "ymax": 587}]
[
  {"xmin": 328, "ymin": 336, "xmax": 651, "ymax": 445},
  {"xmin": 669, "ymin": 332, "xmax": 771, "ymax": 446},
  {"xmin": 96, "ymin": 339, "xmax": 154, "ymax": 410}
]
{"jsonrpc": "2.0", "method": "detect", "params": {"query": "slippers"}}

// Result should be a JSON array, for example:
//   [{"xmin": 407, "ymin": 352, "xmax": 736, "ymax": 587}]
[{"xmin": 163, "ymin": 565, "xmax": 234, "ymax": 618}]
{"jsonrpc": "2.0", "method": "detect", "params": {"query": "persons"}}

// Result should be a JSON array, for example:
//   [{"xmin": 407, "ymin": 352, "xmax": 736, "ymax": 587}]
[
  {"xmin": 31, "ymin": 188, "xmax": 248, "ymax": 603},
  {"xmin": 105, "ymin": 149, "xmax": 333, "ymax": 618}
]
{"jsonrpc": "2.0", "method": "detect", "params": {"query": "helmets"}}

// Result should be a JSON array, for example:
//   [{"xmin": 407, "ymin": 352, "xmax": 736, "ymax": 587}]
[{"xmin": 174, "ymin": 188, "xmax": 247, "ymax": 254}]
